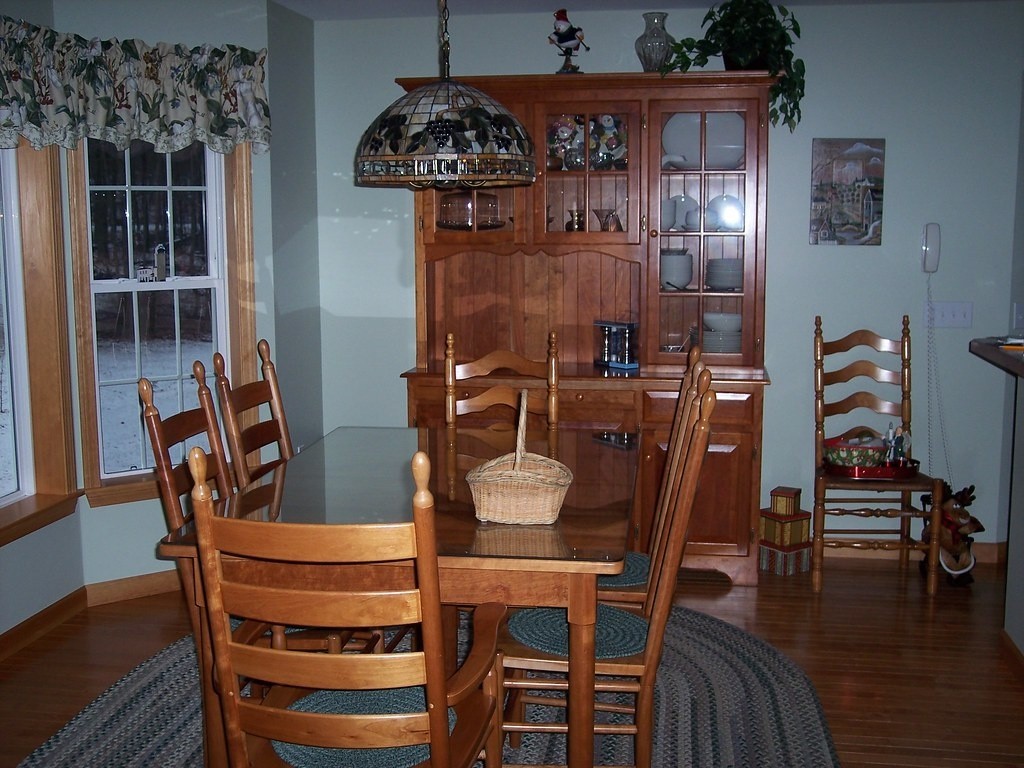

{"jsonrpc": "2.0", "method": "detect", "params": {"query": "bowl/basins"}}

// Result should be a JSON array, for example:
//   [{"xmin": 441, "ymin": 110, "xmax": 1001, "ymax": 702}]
[
  {"xmin": 661, "ymin": 200, "xmax": 675, "ymax": 232},
  {"xmin": 825, "ymin": 444, "xmax": 889, "ymax": 468},
  {"xmin": 703, "ymin": 312, "xmax": 742, "ymax": 331},
  {"xmin": 685, "ymin": 211, "xmax": 718, "ymax": 225},
  {"xmin": 661, "ymin": 246, "xmax": 692, "ymax": 289}
]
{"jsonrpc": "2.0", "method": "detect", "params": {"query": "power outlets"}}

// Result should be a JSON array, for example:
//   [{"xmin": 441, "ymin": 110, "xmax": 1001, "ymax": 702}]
[{"xmin": 923, "ymin": 301, "xmax": 972, "ymax": 328}]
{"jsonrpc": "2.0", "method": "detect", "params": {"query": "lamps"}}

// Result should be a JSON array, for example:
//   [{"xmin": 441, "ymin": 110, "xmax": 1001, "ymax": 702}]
[{"xmin": 354, "ymin": 0, "xmax": 534, "ymax": 192}]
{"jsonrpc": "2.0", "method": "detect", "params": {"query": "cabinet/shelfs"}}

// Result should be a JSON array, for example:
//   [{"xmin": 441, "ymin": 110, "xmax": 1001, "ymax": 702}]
[{"xmin": 394, "ymin": 70, "xmax": 784, "ymax": 588}]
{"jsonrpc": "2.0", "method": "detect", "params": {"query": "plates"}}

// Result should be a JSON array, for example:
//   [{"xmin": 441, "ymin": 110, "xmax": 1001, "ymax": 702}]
[
  {"xmin": 671, "ymin": 195, "xmax": 699, "ymax": 227},
  {"xmin": 701, "ymin": 332, "xmax": 741, "ymax": 353},
  {"xmin": 707, "ymin": 195, "xmax": 744, "ymax": 230},
  {"xmin": 705, "ymin": 259, "xmax": 744, "ymax": 290},
  {"xmin": 661, "ymin": 113, "xmax": 746, "ymax": 170},
  {"xmin": 682, "ymin": 225, "xmax": 721, "ymax": 231}
]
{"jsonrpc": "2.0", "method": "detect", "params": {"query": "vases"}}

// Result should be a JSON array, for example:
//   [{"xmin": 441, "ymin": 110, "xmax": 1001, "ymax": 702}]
[{"xmin": 634, "ymin": 13, "xmax": 677, "ymax": 73}]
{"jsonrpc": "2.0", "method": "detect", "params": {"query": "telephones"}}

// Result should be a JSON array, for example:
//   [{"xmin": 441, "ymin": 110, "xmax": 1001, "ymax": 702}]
[{"xmin": 921, "ymin": 223, "xmax": 941, "ymax": 273}]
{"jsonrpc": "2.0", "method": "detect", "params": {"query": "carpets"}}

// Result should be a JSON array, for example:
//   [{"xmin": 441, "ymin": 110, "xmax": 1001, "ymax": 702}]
[{"xmin": 15, "ymin": 602, "xmax": 841, "ymax": 768}]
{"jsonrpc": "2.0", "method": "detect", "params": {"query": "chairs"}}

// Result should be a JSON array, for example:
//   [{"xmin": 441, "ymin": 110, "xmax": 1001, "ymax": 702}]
[
  {"xmin": 213, "ymin": 338, "xmax": 294, "ymax": 490},
  {"xmin": 598, "ymin": 346, "xmax": 705, "ymax": 612},
  {"xmin": 498, "ymin": 370, "xmax": 718, "ymax": 768},
  {"xmin": 444, "ymin": 331, "xmax": 559, "ymax": 429},
  {"xmin": 138, "ymin": 361, "xmax": 383, "ymax": 768},
  {"xmin": 188, "ymin": 448, "xmax": 509, "ymax": 768},
  {"xmin": 814, "ymin": 315, "xmax": 942, "ymax": 595}
]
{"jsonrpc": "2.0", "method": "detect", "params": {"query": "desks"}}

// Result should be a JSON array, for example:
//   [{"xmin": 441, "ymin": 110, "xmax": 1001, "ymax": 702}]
[{"xmin": 160, "ymin": 426, "xmax": 642, "ymax": 768}]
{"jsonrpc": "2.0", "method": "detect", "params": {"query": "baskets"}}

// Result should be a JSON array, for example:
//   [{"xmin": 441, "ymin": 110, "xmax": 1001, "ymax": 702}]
[{"xmin": 463, "ymin": 388, "xmax": 572, "ymax": 525}]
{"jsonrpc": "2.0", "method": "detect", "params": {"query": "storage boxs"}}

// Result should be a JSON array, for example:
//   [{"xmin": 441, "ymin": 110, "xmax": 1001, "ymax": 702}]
[
  {"xmin": 760, "ymin": 541, "xmax": 812, "ymax": 577},
  {"xmin": 770, "ymin": 486, "xmax": 801, "ymax": 515},
  {"xmin": 760, "ymin": 507, "xmax": 812, "ymax": 546}
]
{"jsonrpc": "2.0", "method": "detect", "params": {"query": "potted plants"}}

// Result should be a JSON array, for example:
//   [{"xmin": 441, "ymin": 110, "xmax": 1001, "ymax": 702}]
[{"xmin": 658, "ymin": 0, "xmax": 806, "ymax": 133}]
